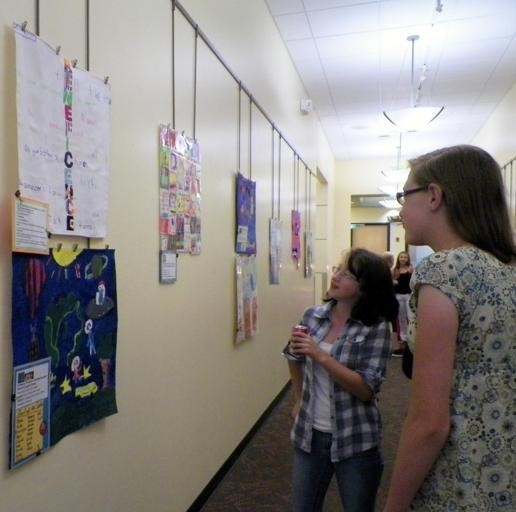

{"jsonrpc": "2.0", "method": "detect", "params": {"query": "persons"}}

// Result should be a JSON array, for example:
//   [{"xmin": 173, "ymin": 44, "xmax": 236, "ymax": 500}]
[
  {"xmin": 383, "ymin": 253, "xmax": 404, "ymax": 358},
  {"xmin": 381, "ymin": 144, "xmax": 516, "ymax": 510},
  {"xmin": 394, "ymin": 251, "xmax": 414, "ymax": 344},
  {"xmin": 280, "ymin": 248, "xmax": 400, "ymax": 512}
]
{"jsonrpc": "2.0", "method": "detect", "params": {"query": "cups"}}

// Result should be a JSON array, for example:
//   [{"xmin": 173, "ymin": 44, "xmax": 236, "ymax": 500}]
[
  {"xmin": 332, "ymin": 264, "xmax": 359, "ymax": 282},
  {"xmin": 397, "ymin": 187, "xmax": 427, "ymax": 205}
]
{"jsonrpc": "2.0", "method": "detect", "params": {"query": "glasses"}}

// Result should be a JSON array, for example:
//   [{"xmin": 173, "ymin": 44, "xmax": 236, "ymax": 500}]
[{"xmin": 382, "ymin": 35, "xmax": 444, "ymax": 133}]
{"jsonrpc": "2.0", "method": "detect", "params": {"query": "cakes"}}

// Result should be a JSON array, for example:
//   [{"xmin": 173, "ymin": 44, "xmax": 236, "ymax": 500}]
[{"xmin": 293, "ymin": 325, "xmax": 308, "ymax": 356}]
